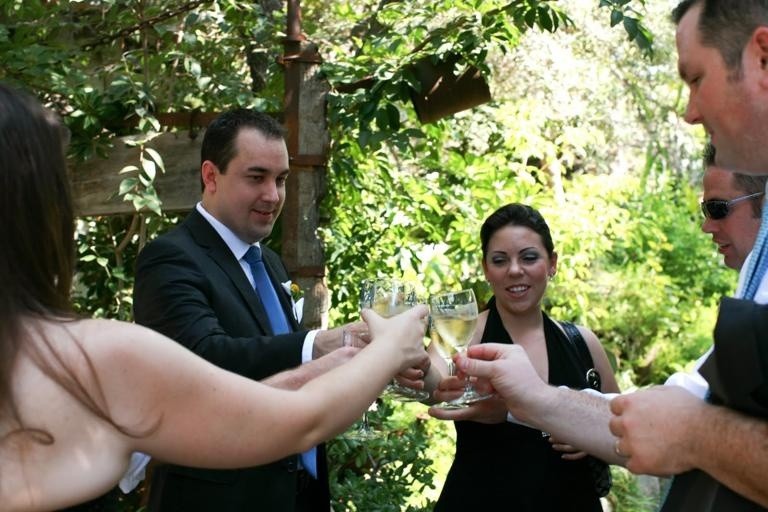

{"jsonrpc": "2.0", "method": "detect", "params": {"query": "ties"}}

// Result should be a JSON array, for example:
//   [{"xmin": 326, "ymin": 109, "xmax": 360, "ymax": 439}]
[
  {"xmin": 243, "ymin": 246, "xmax": 318, "ymax": 483},
  {"xmin": 739, "ymin": 196, "xmax": 768, "ymax": 301}
]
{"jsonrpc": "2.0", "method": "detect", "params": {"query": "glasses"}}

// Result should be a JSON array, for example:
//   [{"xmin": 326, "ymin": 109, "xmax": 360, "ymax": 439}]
[{"xmin": 701, "ymin": 191, "xmax": 765, "ymax": 220}]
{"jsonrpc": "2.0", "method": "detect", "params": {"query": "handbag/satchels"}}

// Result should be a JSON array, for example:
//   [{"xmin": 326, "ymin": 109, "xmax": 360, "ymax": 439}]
[{"xmin": 563, "ymin": 322, "xmax": 612, "ymax": 497}]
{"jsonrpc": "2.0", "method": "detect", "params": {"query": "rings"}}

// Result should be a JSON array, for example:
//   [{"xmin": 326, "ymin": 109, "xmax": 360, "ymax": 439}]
[{"xmin": 615, "ymin": 439, "xmax": 624, "ymax": 457}]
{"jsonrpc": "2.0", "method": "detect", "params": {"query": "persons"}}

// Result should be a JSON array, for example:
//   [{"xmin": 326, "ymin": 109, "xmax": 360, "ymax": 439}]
[
  {"xmin": 0, "ymin": 82, "xmax": 429, "ymax": 512},
  {"xmin": 428, "ymin": 140, "xmax": 768, "ymax": 432},
  {"xmin": 456, "ymin": 1, "xmax": 768, "ymax": 512},
  {"xmin": 133, "ymin": 108, "xmax": 371, "ymax": 512},
  {"xmin": 397, "ymin": 205, "xmax": 621, "ymax": 511}
]
